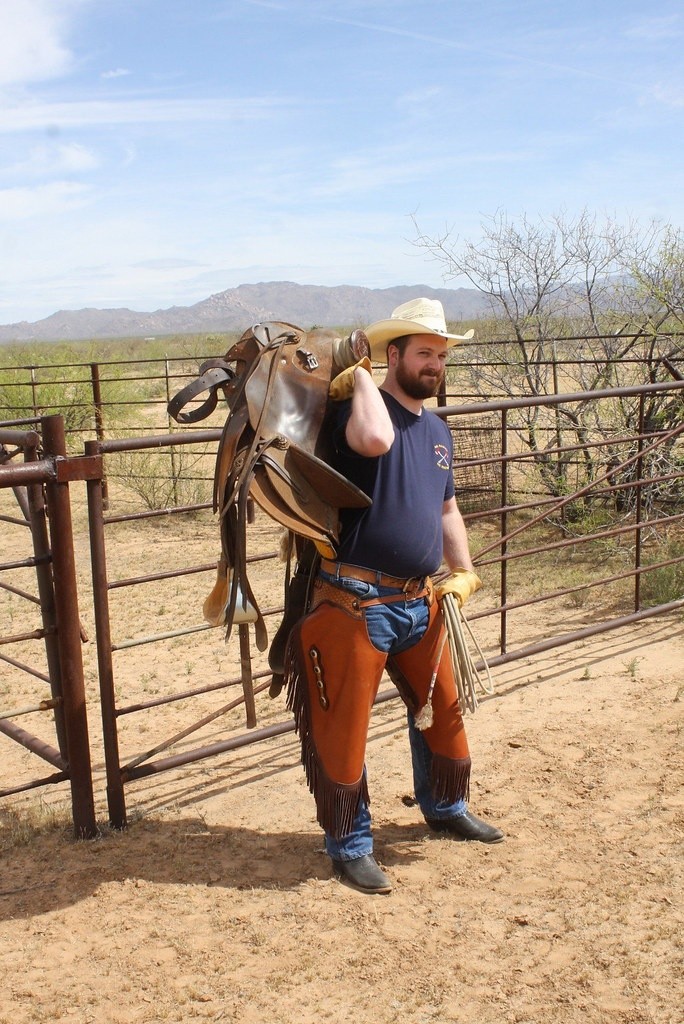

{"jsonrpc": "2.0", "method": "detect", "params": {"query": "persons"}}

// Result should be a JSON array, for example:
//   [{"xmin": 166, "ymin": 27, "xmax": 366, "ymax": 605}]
[{"xmin": 283, "ymin": 298, "xmax": 507, "ymax": 891}]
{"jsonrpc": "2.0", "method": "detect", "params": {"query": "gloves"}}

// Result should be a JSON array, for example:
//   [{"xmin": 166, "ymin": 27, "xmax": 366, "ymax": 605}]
[
  {"xmin": 328, "ymin": 355, "xmax": 373, "ymax": 401},
  {"xmin": 432, "ymin": 566, "xmax": 483, "ymax": 610}
]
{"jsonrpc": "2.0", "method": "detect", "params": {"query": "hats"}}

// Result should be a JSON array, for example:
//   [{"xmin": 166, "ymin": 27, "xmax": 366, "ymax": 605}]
[{"xmin": 362, "ymin": 297, "xmax": 475, "ymax": 364}]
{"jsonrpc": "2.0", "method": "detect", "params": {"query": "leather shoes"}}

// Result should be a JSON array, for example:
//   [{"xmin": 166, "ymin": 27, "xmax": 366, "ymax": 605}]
[
  {"xmin": 332, "ymin": 853, "xmax": 393, "ymax": 893},
  {"xmin": 424, "ymin": 810, "xmax": 506, "ymax": 844}
]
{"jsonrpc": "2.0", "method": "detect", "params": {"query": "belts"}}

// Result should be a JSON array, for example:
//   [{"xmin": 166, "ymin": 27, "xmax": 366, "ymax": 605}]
[{"xmin": 318, "ymin": 557, "xmax": 424, "ymax": 594}]
